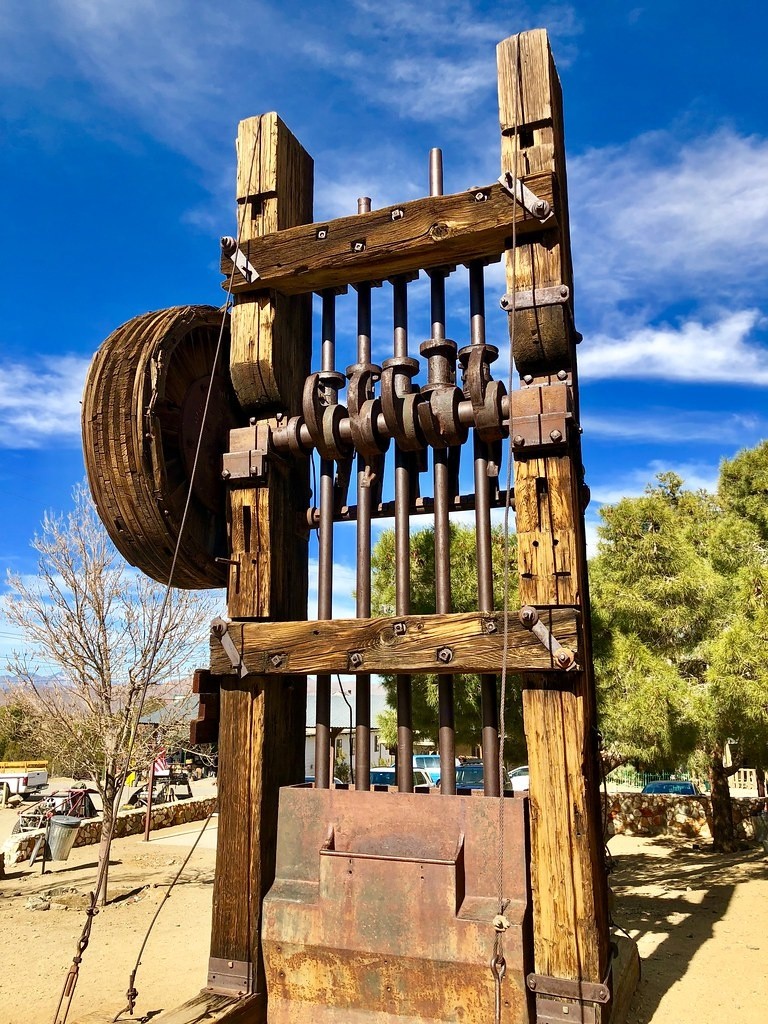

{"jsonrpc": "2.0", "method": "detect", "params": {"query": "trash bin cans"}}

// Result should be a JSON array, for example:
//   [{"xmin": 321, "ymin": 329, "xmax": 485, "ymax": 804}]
[{"xmin": 48, "ymin": 815, "xmax": 80, "ymax": 862}]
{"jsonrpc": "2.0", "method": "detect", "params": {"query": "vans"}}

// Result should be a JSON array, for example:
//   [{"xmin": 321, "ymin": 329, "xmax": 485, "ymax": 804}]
[{"xmin": 391, "ymin": 755, "xmax": 461, "ymax": 785}]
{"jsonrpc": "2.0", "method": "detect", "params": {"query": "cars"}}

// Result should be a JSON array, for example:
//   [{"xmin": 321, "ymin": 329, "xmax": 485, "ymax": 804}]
[
  {"xmin": 368, "ymin": 768, "xmax": 435, "ymax": 788},
  {"xmin": 641, "ymin": 781, "xmax": 706, "ymax": 796},
  {"xmin": 508, "ymin": 766, "xmax": 529, "ymax": 792},
  {"xmin": 436, "ymin": 765, "xmax": 513, "ymax": 790}
]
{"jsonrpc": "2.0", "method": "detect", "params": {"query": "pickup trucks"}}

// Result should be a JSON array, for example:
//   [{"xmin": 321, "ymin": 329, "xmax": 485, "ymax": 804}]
[{"xmin": 0, "ymin": 771, "xmax": 49, "ymax": 803}]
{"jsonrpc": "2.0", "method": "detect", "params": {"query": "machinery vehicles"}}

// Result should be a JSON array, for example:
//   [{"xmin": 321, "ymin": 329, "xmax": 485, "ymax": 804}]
[{"xmin": 11, "ymin": 788, "xmax": 103, "ymax": 835}]
